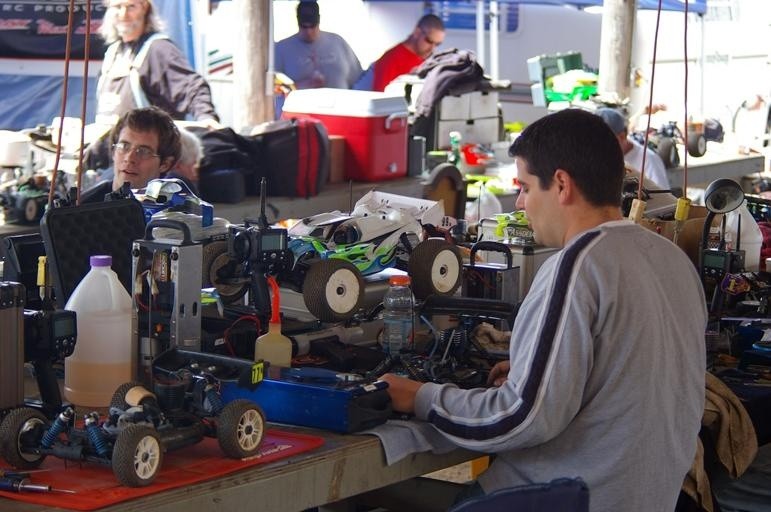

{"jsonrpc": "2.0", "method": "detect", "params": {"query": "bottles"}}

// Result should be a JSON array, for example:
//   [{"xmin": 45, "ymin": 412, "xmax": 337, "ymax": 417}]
[
  {"xmin": 59, "ymin": 250, "xmax": 135, "ymax": 411},
  {"xmin": 380, "ymin": 272, "xmax": 417, "ymax": 359},
  {"xmin": 252, "ymin": 318, "xmax": 295, "ymax": 369}
]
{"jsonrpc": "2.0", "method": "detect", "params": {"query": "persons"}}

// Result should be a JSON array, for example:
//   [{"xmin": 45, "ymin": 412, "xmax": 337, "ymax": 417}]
[
  {"xmin": 371, "ymin": 109, "xmax": 709, "ymax": 512},
  {"xmin": 93, "ymin": 0, "xmax": 221, "ymax": 129},
  {"xmin": 592, "ymin": 107, "xmax": 672, "ymax": 194},
  {"xmin": 352, "ymin": 14, "xmax": 447, "ymax": 92},
  {"xmin": 36, "ymin": 105, "xmax": 201, "ymax": 305},
  {"xmin": 272, "ymin": 1, "xmax": 364, "ymax": 121}
]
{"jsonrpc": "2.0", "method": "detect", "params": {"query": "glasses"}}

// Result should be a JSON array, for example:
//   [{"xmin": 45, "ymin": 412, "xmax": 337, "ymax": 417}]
[
  {"xmin": 112, "ymin": 143, "xmax": 161, "ymax": 159},
  {"xmin": 419, "ymin": 26, "xmax": 441, "ymax": 47},
  {"xmin": 299, "ymin": 23, "xmax": 318, "ymax": 28}
]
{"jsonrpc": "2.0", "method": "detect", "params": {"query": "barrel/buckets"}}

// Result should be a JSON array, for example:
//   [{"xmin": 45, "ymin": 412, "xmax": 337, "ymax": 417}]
[{"xmin": 64, "ymin": 256, "xmax": 138, "ymax": 406}]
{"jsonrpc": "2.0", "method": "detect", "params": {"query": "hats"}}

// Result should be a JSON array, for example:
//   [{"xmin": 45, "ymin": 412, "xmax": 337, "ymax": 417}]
[
  {"xmin": 297, "ymin": 2, "xmax": 318, "ymax": 23},
  {"xmin": 592, "ymin": 107, "xmax": 625, "ymax": 136}
]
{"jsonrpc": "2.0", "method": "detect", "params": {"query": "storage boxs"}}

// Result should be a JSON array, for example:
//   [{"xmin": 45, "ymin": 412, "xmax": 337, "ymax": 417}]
[{"xmin": 282, "ymin": 87, "xmax": 411, "ymax": 183}]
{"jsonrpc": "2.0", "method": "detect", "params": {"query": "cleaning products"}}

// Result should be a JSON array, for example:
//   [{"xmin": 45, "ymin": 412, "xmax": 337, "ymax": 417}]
[{"xmin": 447, "ymin": 131, "xmax": 466, "ymax": 176}]
